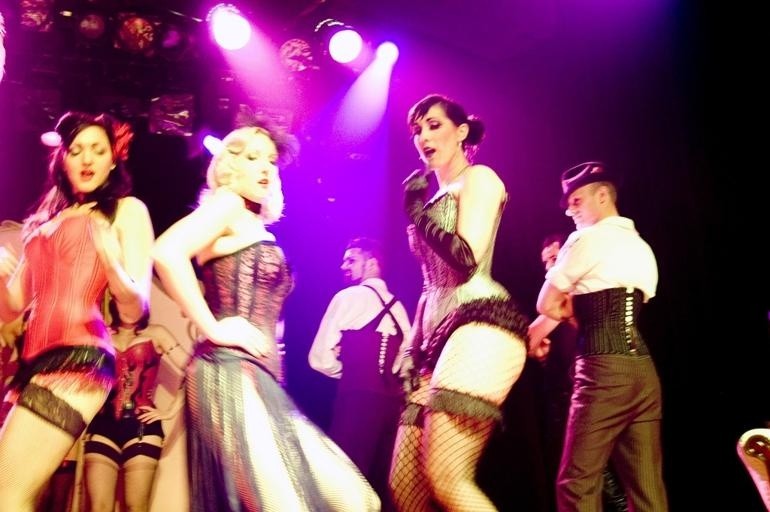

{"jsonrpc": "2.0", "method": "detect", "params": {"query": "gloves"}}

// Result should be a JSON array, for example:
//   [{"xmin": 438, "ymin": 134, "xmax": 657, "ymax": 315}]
[{"xmin": 405, "ymin": 177, "xmax": 476, "ymax": 272}]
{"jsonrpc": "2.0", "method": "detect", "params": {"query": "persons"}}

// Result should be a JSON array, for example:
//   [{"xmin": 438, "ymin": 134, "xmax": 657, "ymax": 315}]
[
  {"xmin": 390, "ymin": 95, "xmax": 531, "ymax": 511},
  {"xmin": 308, "ymin": 237, "xmax": 412, "ymax": 512},
  {"xmin": 0, "ymin": 110, "xmax": 381, "ymax": 512},
  {"xmin": 528, "ymin": 162, "xmax": 668, "ymax": 511}
]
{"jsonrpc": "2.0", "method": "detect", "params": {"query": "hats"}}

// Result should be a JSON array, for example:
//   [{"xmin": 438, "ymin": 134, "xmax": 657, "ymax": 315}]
[{"xmin": 560, "ymin": 162, "xmax": 616, "ymax": 207}]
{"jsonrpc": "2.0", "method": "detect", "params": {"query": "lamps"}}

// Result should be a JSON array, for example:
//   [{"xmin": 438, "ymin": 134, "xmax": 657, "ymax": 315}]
[{"xmin": 20, "ymin": 0, "xmax": 364, "ymax": 74}]
